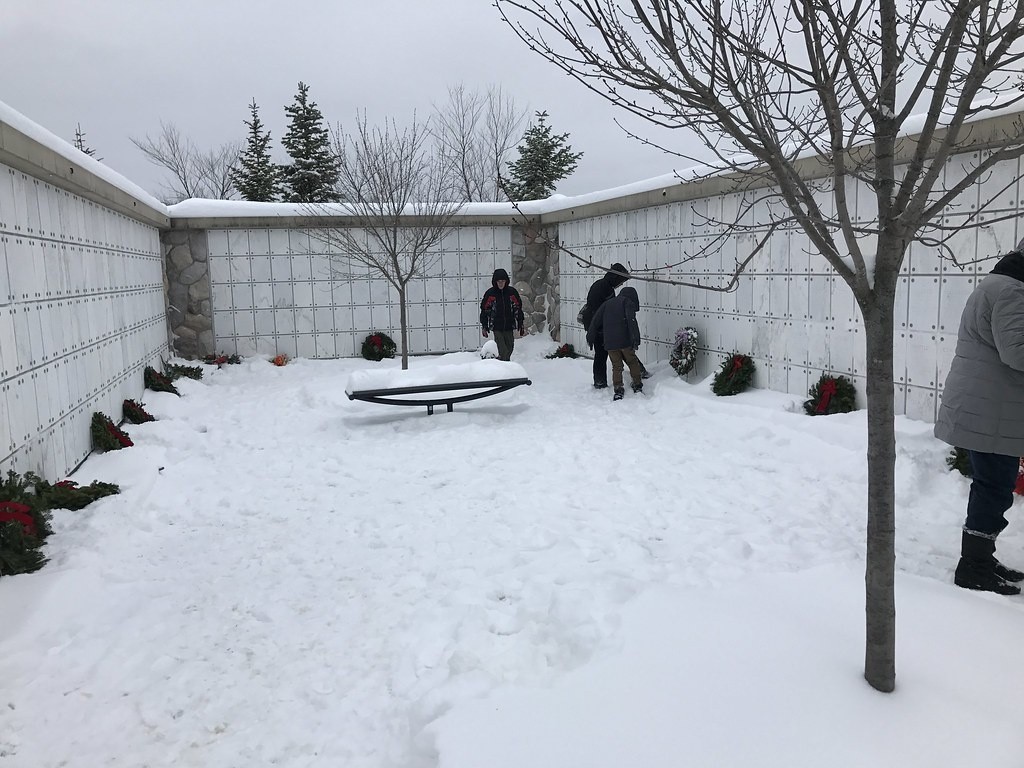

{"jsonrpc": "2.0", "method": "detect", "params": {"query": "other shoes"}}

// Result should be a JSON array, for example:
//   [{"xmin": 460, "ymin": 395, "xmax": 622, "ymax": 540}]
[
  {"xmin": 633, "ymin": 383, "xmax": 643, "ymax": 393},
  {"xmin": 613, "ymin": 387, "xmax": 624, "ymax": 400}
]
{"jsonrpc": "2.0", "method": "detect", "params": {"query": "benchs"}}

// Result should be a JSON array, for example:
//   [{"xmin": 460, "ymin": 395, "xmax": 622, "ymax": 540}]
[{"xmin": 344, "ymin": 377, "xmax": 532, "ymax": 416}]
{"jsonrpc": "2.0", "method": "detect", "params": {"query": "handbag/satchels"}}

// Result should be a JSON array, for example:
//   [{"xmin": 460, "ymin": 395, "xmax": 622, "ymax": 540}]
[{"xmin": 577, "ymin": 304, "xmax": 591, "ymax": 325}]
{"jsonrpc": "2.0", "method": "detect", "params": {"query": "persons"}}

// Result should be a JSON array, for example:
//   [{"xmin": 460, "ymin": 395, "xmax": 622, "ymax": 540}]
[
  {"xmin": 578, "ymin": 263, "xmax": 654, "ymax": 389},
  {"xmin": 586, "ymin": 287, "xmax": 643, "ymax": 401},
  {"xmin": 479, "ymin": 269, "xmax": 525, "ymax": 361},
  {"xmin": 934, "ymin": 235, "xmax": 1024, "ymax": 595}
]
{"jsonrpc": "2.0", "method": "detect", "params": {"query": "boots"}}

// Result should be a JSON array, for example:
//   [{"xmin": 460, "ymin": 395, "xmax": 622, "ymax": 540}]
[{"xmin": 954, "ymin": 518, "xmax": 1024, "ymax": 595}]
